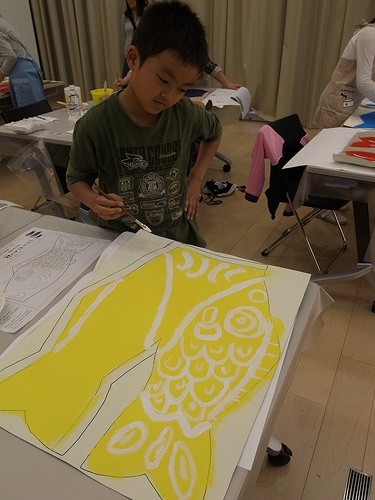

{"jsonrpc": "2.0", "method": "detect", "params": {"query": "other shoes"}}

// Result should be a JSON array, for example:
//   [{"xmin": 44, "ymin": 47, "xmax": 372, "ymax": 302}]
[{"xmin": 203, "ymin": 179, "xmax": 238, "ymax": 196}]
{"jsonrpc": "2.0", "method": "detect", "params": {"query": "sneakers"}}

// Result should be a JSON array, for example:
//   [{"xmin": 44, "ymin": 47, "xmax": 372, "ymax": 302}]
[{"xmin": 316, "ymin": 209, "xmax": 347, "ymax": 224}]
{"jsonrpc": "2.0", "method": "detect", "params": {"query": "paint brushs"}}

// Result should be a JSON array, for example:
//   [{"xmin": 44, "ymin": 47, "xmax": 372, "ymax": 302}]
[
  {"xmin": 116, "ymin": 72, "xmax": 120, "ymax": 91},
  {"xmin": 92, "ymin": 182, "xmax": 153, "ymax": 233}
]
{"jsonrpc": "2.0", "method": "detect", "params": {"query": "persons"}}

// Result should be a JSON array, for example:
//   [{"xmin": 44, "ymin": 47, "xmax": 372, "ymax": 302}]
[
  {"xmin": 122, "ymin": 0, "xmax": 148, "ymax": 89},
  {"xmin": 67, "ymin": 1, "xmax": 222, "ymax": 248},
  {"xmin": 0, "ymin": 20, "xmax": 53, "ymax": 120},
  {"xmin": 113, "ymin": 59, "xmax": 241, "ymax": 90},
  {"xmin": 312, "ymin": 19, "xmax": 375, "ymax": 225}
]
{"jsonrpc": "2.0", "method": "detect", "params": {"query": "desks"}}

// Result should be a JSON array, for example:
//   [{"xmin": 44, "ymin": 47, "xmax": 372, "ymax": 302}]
[{"xmin": 0, "ymin": 79, "xmax": 375, "ymax": 500}]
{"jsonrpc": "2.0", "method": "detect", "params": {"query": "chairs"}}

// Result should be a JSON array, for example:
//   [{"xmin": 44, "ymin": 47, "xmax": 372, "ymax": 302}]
[{"xmin": 244, "ymin": 114, "xmax": 352, "ymax": 275}]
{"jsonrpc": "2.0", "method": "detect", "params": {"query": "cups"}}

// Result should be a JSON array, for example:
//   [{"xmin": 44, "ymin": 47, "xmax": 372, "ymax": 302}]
[{"xmin": 90, "ymin": 88, "xmax": 113, "ymax": 104}]
